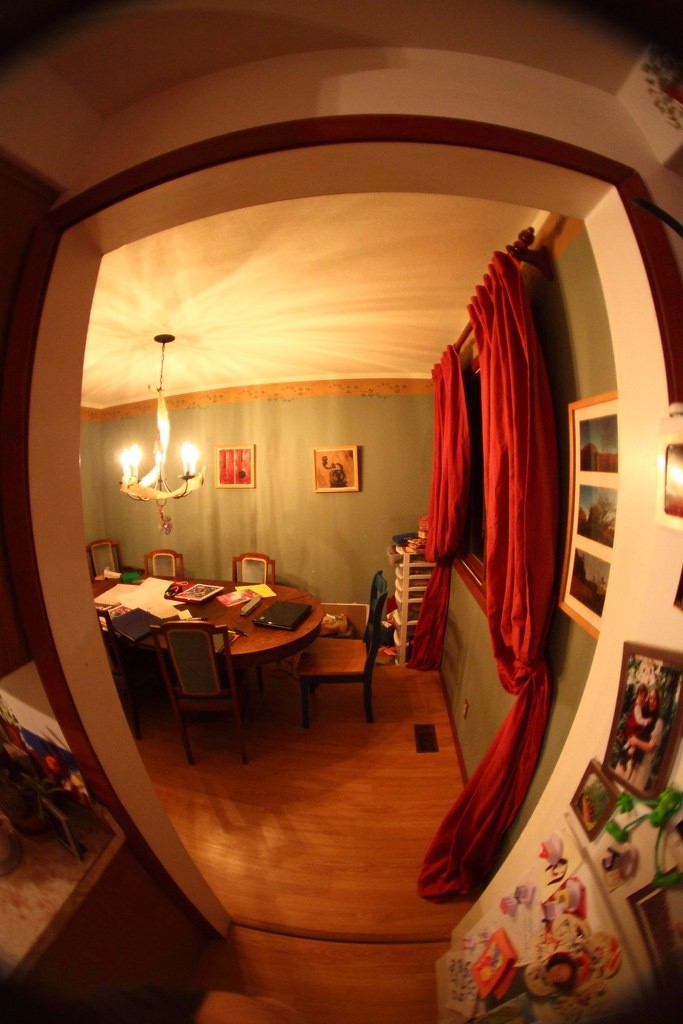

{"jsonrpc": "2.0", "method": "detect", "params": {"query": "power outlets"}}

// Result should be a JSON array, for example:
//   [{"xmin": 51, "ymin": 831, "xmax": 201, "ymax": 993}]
[{"xmin": 463, "ymin": 699, "xmax": 468, "ymax": 721}]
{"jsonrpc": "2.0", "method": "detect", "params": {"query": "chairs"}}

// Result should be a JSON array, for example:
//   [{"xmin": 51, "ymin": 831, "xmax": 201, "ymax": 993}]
[
  {"xmin": 86, "ymin": 540, "xmax": 123, "ymax": 576},
  {"xmin": 232, "ymin": 553, "xmax": 275, "ymax": 584},
  {"xmin": 296, "ymin": 569, "xmax": 388, "ymax": 729},
  {"xmin": 144, "ymin": 549, "xmax": 184, "ymax": 577},
  {"xmin": 149, "ymin": 621, "xmax": 247, "ymax": 765},
  {"xmin": 97, "ymin": 611, "xmax": 166, "ymax": 740}
]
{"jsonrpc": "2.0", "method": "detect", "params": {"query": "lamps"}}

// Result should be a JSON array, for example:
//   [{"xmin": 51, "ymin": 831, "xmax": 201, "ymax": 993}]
[{"xmin": 119, "ymin": 333, "xmax": 204, "ymax": 507}]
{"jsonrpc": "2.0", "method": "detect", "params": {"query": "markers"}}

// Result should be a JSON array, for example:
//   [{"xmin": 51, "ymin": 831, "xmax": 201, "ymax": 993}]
[{"xmin": 189, "ymin": 617, "xmax": 208, "ymax": 620}]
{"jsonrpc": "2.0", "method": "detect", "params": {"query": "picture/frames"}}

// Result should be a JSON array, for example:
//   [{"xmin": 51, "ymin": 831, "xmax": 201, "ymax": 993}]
[
  {"xmin": 657, "ymin": 436, "xmax": 683, "ymax": 532},
  {"xmin": 311, "ymin": 445, "xmax": 359, "ymax": 493},
  {"xmin": 569, "ymin": 759, "xmax": 621, "ymax": 843},
  {"xmin": 215, "ymin": 443, "xmax": 255, "ymax": 489},
  {"xmin": 175, "ymin": 584, "xmax": 224, "ymax": 601},
  {"xmin": 601, "ymin": 641, "xmax": 683, "ymax": 811},
  {"xmin": 625, "ymin": 866, "xmax": 683, "ymax": 1005},
  {"xmin": 558, "ymin": 388, "xmax": 618, "ymax": 639}
]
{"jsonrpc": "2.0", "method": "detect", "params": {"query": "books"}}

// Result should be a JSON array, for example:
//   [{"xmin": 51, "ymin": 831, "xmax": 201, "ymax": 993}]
[{"xmin": 94, "ymin": 569, "xmax": 313, "ymax": 642}]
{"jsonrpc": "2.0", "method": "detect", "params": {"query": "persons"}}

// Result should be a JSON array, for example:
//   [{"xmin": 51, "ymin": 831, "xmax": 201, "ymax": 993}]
[
  {"xmin": 614, "ymin": 687, "xmax": 666, "ymax": 792},
  {"xmin": 619, "ymin": 684, "xmax": 653, "ymax": 772}
]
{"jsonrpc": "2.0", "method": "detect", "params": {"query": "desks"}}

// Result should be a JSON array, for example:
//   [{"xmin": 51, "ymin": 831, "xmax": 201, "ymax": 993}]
[{"xmin": 92, "ymin": 575, "xmax": 324, "ymax": 690}]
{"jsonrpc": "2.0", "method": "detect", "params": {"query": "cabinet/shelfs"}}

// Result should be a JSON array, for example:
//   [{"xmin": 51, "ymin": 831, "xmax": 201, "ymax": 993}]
[{"xmin": 394, "ymin": 545, "xmax": 437, "ymax": 666}]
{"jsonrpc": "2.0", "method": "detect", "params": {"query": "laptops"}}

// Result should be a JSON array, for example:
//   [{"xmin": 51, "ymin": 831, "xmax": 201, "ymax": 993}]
[{"xmin": 252, "ymin": 601, "xmax": 312, "ymax": 630}]
